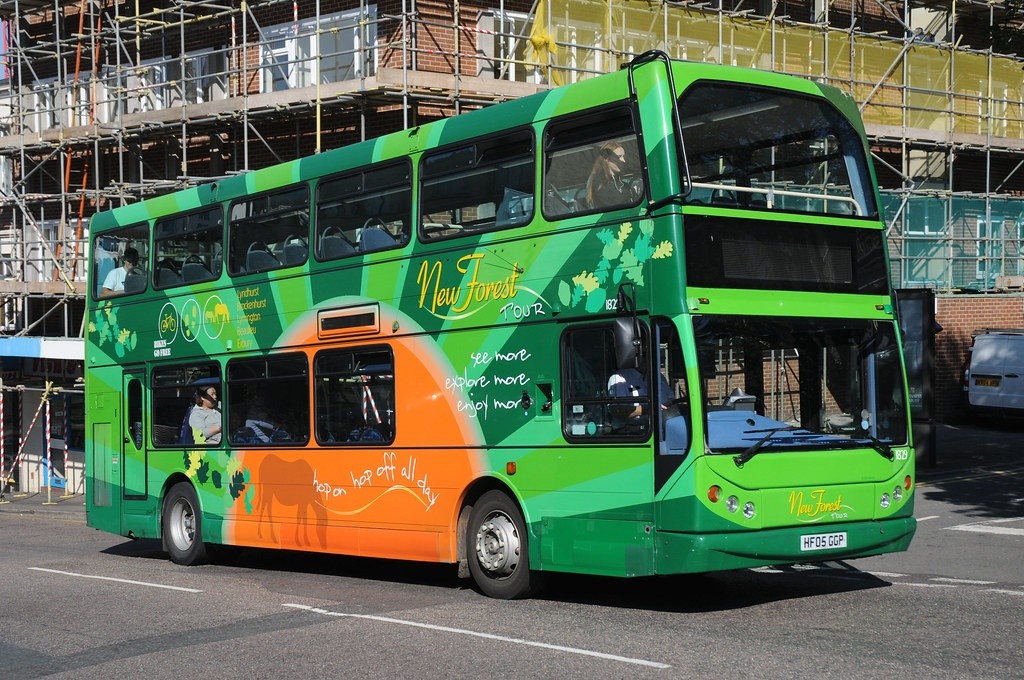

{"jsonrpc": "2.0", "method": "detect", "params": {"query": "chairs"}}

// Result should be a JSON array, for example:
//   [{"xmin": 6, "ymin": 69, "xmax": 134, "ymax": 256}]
[
  {"xmin": 97, "ymin": 216, "xmax": 397, "ymax": 299},
  {"xmin": 547, "ymin": 184, "xmax": 774, "ymax": 217},
  {"xmin": 180, "ymin": 388, "xmax": 387, "ymax": 443}
]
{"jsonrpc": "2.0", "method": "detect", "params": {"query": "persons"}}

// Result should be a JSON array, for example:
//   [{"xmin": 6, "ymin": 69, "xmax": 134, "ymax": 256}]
[
  {"xmin": 608, "ymin": 348, "xmax": 675, "ymax": 416},
  {"xmin": 180, "ymin": 386, "xmax": 222, "ymax": 445},
  {"xmin": 587, "ymin": 141, "xmax": 631, "ymax": 209},
  {"xmin": 100, "ymin": 247, "xmax": 138, "ymax": 297}
]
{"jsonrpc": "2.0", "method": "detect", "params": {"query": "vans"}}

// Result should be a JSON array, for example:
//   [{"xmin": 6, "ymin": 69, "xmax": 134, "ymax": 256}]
[{"xmin": 960, "ymin": 331, "xmax": 1024, "ymax": 427}]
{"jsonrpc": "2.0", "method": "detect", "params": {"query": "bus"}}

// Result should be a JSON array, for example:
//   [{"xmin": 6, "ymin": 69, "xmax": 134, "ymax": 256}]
[{"xmin": 85, "ymin": 50, "xmax": 917, "ymax": 599}]
